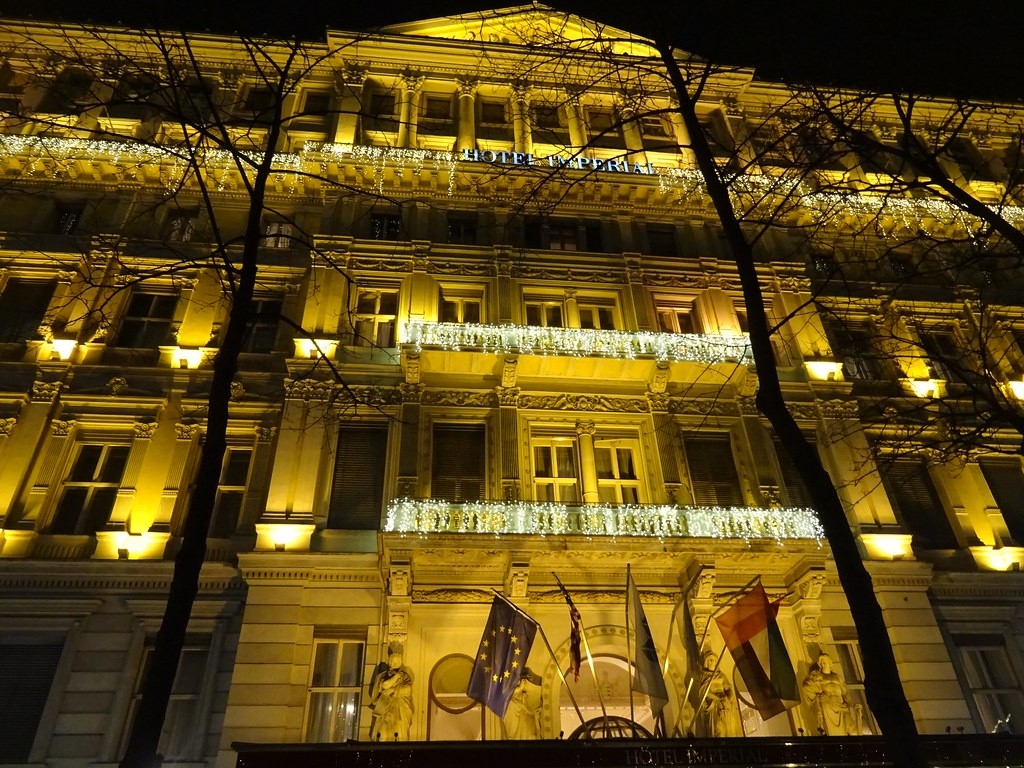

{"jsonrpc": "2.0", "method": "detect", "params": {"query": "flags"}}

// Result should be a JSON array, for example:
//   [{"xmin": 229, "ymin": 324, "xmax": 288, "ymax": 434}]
[
  {"xmin": 714, "ymin": 582, "xmax": 801, "ymax": 722},
  {"xmin": 467, "ymin": 596, "xmax": 538, "ymax": 719},
  {"xmin": 626, "ymin": 573, "xmax": 669, "ymax": 720},
  {"xmin": 557, "ymin": 582, "xmax": 583, "ymax": 683}
]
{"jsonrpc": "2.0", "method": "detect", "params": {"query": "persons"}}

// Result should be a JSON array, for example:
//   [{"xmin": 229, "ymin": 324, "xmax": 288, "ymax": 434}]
[
  {"xmin": 699, "ymin": 649, "xmax": 736, "ymax": 738},
  {"xmin": 802, "ymin": 654, "xmax": 863, "ymax": 736},
  {"xmin": 371, "ymin": 653, "xmax": 414, "ymax": 741}
]
